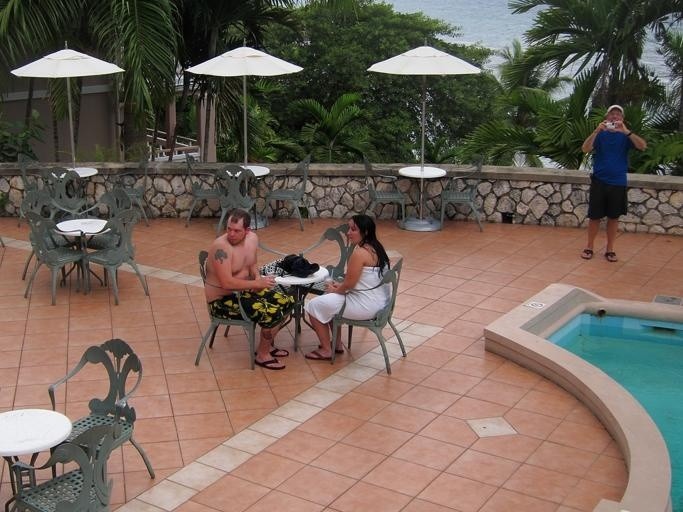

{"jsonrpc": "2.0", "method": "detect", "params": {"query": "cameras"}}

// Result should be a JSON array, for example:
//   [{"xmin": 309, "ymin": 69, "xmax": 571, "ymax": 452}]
[{"xmin": 605, "ymin": 122, "xmax": 615, "ymax": 129}]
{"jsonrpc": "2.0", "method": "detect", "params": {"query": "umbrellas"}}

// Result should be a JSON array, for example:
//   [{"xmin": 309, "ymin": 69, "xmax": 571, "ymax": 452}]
[
  {"xmin": 366, "ymin": 35, "xmax": 480, "ymax": 233},
  {"xmin": 9, "ymin": 41, "xmax": 126, "ymax": 186},
  {"xmin": 184, "ymin": 37, "xmax": 305, "ymax": 230}
]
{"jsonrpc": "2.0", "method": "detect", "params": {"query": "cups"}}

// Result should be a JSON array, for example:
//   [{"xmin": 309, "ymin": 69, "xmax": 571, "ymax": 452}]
[{"xmin": 265, "ymin": 272, "xmax": 275, "ymax": 290}]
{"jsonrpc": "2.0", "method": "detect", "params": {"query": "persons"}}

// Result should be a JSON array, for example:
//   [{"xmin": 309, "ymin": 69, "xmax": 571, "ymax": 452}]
[
  {"xmin": 303, "ymin": 214, "xmax": 393, "ymax": 361},
  {"xmin": 204, "ymin": 208, "xmax": 296, "ymax": 371},
  {"xmin": 581, "ymin": 104, "xmax": 646, "ymax": 262}
]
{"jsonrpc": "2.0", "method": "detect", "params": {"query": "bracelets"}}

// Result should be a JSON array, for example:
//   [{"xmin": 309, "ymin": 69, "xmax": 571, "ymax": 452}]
[{"xmin": 625, "ymin": 132, "xmax": 632, "ymax": 137}]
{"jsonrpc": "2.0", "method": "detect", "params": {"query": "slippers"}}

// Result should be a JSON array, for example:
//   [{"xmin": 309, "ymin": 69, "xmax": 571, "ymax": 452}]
[
  {"xmin": 304, "ymin": 350, "xmax": 332, "ymax": 361},
  {"xmin": 579, "ymin": 247, "xmax": 594, "ymax": 260},
  {"xmin": 318, "ymin": 341, "xmax": 346, "ymax": 355},
  {"xmin": 605, "ymin": 248, "xmax": 618, "ymax": 263},
  {"xmin": 254, "ymin": 345, "xmax": 290, "ymax": 356},
  {"xmin": 253, "ymin": 356, "xmax": 286, "ymax": 370}
]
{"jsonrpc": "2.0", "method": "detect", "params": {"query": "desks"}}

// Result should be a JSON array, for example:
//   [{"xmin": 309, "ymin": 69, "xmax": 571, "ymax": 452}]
[{"xmin": 398, "ymin": 166, "xmax": 446, "ymax": 232}]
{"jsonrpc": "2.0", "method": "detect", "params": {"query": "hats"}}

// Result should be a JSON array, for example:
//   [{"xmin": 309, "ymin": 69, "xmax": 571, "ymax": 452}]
[{"xmin": 604, "ymin": 104, "xmax": 626, "ymax": 119}]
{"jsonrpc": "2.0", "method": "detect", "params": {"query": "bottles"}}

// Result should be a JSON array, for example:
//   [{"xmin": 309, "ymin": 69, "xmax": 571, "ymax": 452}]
[{"xmin": 298, "ymin": 248, "xmax": 303, "ymax": 256}]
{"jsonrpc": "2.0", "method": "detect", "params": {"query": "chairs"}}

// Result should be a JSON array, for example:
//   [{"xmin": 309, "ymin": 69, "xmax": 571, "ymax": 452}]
[
  {"xmin": 195, "ymin": 224, "xmax": 408, "ymax": 375},
  {"xmin": 186, "ymin": 154, "xmax": 313, "ymax": 239},
  {"xmin": 362, "ymin": 154, "xmax": 406, "ymax": 229},
  {"xmin": 0, "ymin": 340, "xmax": 156, "ymax": 512},
  {"xmin": 14, "ymin": 152, "xmax": 157, "ymax": 306},
  {"xmin": 440, "ymin": 156, "xmax": 486, "ymax": 233}
]
